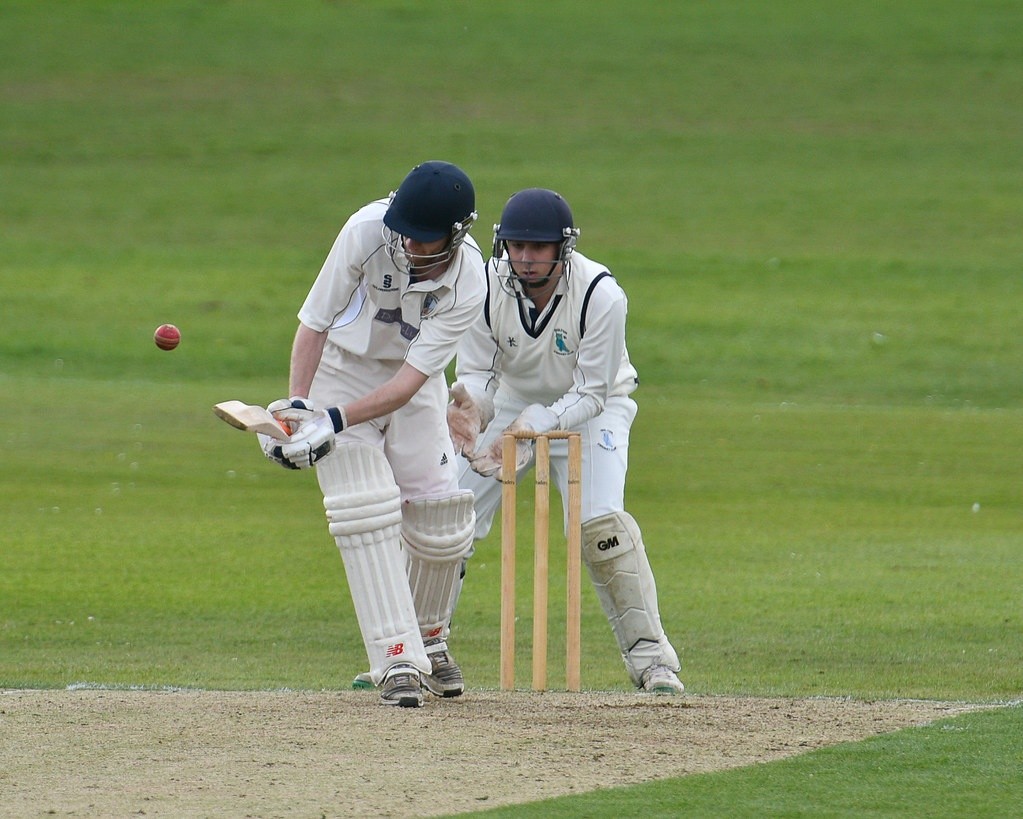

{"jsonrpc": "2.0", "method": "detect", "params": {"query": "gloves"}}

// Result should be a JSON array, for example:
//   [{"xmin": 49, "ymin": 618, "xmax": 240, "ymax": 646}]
[
  {"xmin": 264, "ymin": 406, "xmax": 346, "ymax": 470},
  {"xmin": 471, "ymin": 403, "xmax": 559, "ymax": 481},
  {"xmin": 447, "ymin": 381, "xmax": 495, "ymax": 456},
  {"xmin": 256, "ymin": 397, "xmax": 314, "ymax": 454}
]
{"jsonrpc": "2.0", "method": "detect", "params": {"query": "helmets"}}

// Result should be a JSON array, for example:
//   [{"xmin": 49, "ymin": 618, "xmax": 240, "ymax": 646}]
[
  {"xmin": 496, "ymin": 188, "xmax": 574, "ymax": 242},
  {"xmin": 384, "ymin": 160, "xmax": 476, "ymax": 242}
]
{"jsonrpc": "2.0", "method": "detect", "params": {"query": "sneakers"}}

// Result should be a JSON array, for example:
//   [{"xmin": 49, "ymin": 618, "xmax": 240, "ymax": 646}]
[
  {"xmin": 420, "ymin": 640, "xmax": 464, "ymax": 697},
  {"xmin": 353, "ymin": 672, "xmax": 372, "ymax": 687},
  {"xmin": 380, "ymin": 664, "xmax": 424, "ymax": 707},
  {"xmin": 641, "ymin": 665, "xmax": 685, "ymax": 695}
]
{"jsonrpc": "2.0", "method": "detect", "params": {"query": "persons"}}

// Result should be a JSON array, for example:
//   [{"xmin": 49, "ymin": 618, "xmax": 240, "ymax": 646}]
[
  {"xmin": 353, "ymin": 189, "xmax": 685, "ymax": 693},
  {"xmin": 258, "ymin": 162, "xmax": 489, "ymax": 699}
]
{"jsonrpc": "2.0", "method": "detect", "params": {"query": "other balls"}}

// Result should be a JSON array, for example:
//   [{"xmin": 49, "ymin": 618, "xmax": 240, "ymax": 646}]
[{"xmin": 153, "ymin": 324, "xmax": 181, "ymax": 351}]
